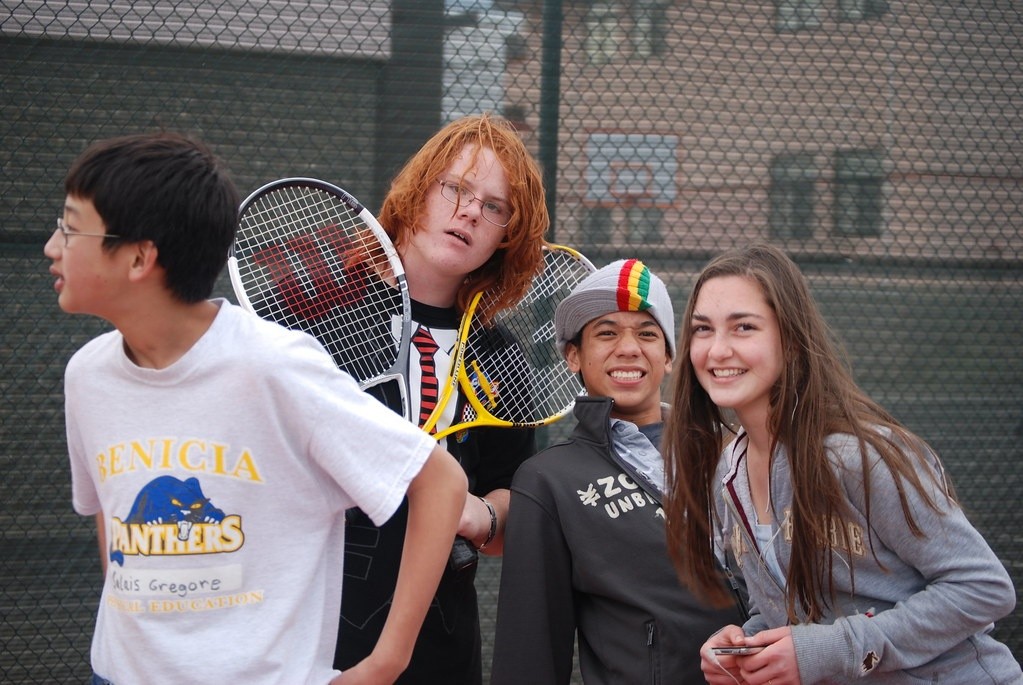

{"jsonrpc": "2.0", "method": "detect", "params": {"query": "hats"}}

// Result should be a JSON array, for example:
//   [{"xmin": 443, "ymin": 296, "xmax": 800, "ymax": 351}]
[{"xmin": 554, "ymin": 258, "xmax": 676, "ymax": 363}]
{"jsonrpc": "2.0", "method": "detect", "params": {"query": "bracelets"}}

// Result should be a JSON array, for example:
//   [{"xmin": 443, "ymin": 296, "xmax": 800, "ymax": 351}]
[{"xmin": 476, "ymin": 497, "xmax": 497, "ymax": 551}]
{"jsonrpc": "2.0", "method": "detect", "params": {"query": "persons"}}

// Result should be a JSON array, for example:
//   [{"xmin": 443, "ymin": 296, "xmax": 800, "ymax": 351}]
[
  {"xmin": 489, "ymin": 258, "xmax": 751, "ymax": 685},
  {"xmin": 661, "ymin": 243, "xmax": 1023, "ymax": 685},
  {"xmin": 255, "ymin": 114, "xmax": 550, "ymax": 685},
  {"xmin": 43, "ymin": 133, "xmax": 468, "ymax": 685}
]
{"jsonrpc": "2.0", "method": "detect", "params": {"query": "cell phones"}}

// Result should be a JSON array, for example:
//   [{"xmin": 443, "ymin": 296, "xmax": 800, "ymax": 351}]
[{"xmin": 711, "ymin": 646, "xmax": 765, "ymax": 656}]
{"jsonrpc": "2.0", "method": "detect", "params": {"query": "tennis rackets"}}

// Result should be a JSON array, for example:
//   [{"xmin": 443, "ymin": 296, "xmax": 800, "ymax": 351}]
[
  {"xmin": 418, "ymin": 240, "xmax": 601, "ymax": 443},
  {"xmin": 223, "ymin": 175, "xmax": 478, "ymax": 575}
]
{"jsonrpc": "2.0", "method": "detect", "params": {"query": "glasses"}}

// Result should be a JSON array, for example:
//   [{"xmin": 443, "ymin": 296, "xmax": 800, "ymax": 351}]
[
  {"xmin": 435, "ymin": 177, "xmax": 513, "ymax": 229},
  {"xmin": 56, "ymin": 218, "xmax": 120, "ymax": 250}
]
